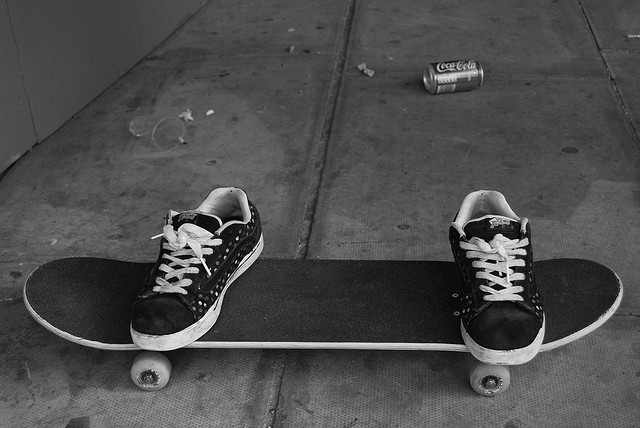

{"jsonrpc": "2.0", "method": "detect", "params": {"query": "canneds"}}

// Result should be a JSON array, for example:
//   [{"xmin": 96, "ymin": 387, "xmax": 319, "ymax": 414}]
[{"xmin": 422, "ymin": 58, "xmax": 484, "ymax": 96}]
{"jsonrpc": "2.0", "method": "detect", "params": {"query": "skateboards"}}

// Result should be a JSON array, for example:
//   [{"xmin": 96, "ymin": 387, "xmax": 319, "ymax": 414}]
[{"xmin": 24, "ymin": 257, "xmax": 624, "ymax": 396}]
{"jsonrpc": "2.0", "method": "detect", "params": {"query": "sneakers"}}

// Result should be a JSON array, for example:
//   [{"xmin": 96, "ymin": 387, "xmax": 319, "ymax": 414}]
[
  {"xmin": 449, "ymin": 188, "xmax": 546, "ymax": 365},
  {"xmin": 129, "ymin": 186, "xmax": 265, "ymax": 351}
]
